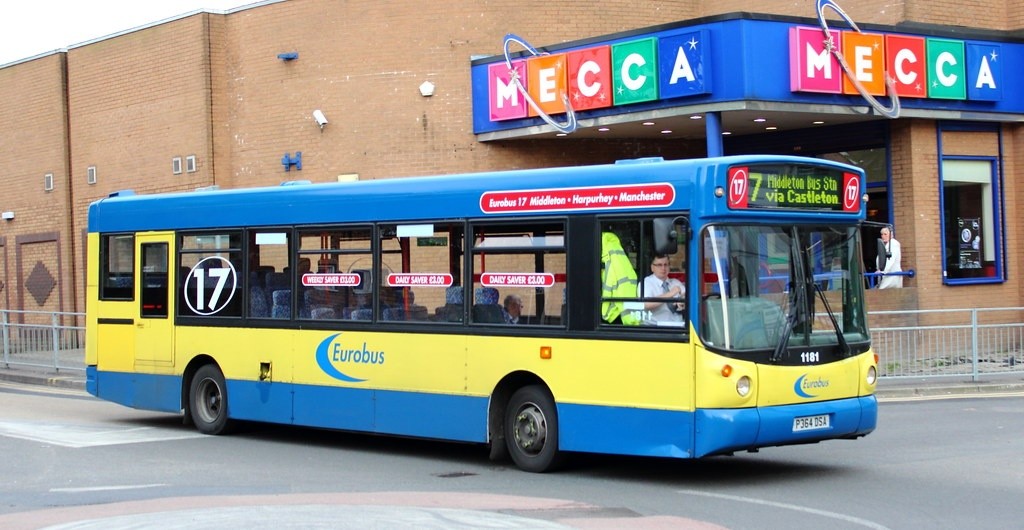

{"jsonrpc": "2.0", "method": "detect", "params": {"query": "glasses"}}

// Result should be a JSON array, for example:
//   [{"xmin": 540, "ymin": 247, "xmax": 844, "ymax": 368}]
[{"xmin": 652, "ymin": 263, "xmax": 668, "ymax": 267}]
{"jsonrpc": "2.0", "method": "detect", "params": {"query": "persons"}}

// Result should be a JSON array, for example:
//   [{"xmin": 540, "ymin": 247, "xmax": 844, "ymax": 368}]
[
  {"xmin": 637, "ymin": 252, "xmax": 686, "ymax": 326},
  {"xmin": 297, "ymin": 258, "xmax": 414, "ymax": 311},
  {"xmin": 502, "ymin": 292, "xmax": 524, "ymax": 324},
  {"xmin": 878, "ymin": 226, "xmax": 904, "ymax": 289}
]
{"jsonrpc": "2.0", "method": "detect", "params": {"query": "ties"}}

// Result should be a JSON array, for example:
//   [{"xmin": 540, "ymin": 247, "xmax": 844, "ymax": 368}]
[{"xmin": 662, "ymin": 282, "xmax": 674, "ymax": 314}]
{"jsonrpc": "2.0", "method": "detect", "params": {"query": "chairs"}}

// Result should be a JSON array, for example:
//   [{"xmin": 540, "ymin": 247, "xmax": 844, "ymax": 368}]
[{"xmin": 116, "ymin": 257, "xmax": 505, "ymax": 323}]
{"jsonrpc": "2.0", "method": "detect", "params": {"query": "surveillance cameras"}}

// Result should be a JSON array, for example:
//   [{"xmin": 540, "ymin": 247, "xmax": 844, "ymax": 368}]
[{"xmin": 313, "ymin": 110, "xmax": 328, "ymax": 126}]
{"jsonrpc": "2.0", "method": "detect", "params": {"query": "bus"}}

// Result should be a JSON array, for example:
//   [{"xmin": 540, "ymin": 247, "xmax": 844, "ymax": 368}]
[{"xmin": 85, "ymin": 154, "xmax": 893, "ymax": 474}]
